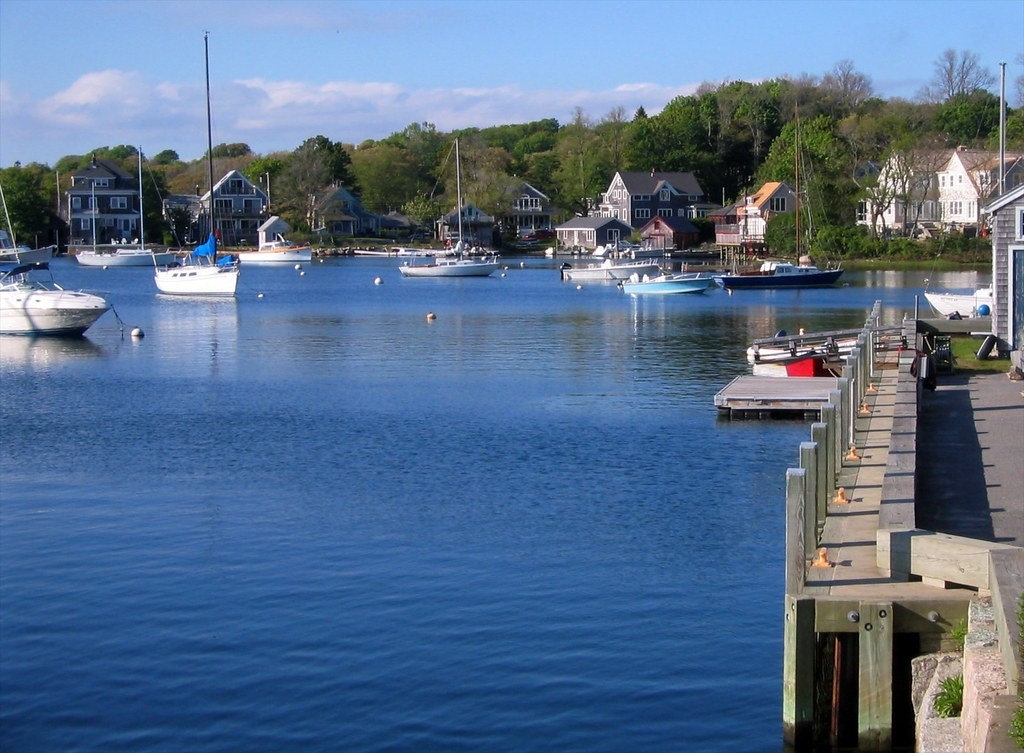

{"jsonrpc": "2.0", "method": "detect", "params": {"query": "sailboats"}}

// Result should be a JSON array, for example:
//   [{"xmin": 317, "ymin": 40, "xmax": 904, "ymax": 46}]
[
  {"xmin": 398, "ymin": 137, "xmax": 501, "ymax": 277},
  {"xmin": 153, "ymin": 31, "xmax": 240, "ymax": 296},
  {"xmin": 75, "ymin": 144, "xmax": 183, "ymax": 266},
  {"xmin": 924, "ymin": 62, "xmax": 1006, "ymax": 318},
  {"xmin": 711, "ymin": 99, "xmax": 846, "ymax": 290}
]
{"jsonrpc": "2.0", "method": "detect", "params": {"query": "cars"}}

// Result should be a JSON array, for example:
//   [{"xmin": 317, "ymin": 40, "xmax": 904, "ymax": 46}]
[{"xmin": 524, "ymin": 230, "xmax": 555, "ymax": 240}]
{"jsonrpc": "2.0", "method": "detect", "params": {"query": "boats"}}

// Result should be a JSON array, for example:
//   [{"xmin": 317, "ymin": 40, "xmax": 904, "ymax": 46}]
[
  {"xmin": 0, "ymin": 183, "xmax": 113, "ymax": 334},
  {"xmin": 591, "ymin": 241, "xmax": 726, "ymax": 260},
  {"xmin": 617, "ymin": 246, "xmax": 712, "ymax": 295},
  {"xmin": 238, "ymin": 241, "xmax": 312, "ymax": 261},
  {"xmin": 560, "ymin": 258, "xmax": 659, "ymax": 281},
  {"xmin": 747, "ymin": 336, "xmax": 902, "ymax": 378},
  {"xmin": 0, "ymin": 230, "xmax": 54, "ymax": 264}
]
